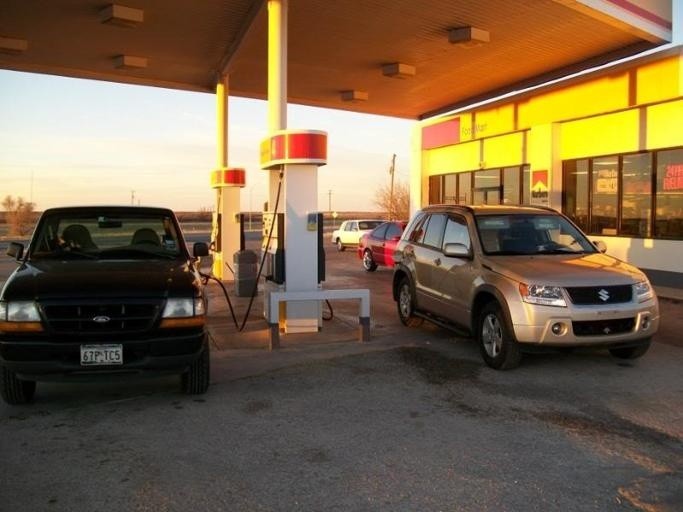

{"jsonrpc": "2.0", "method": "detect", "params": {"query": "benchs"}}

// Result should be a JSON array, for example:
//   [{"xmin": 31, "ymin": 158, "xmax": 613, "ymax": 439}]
[{"xmin": 479, "ymin": 228, "xmax": 501, "ymax": 253}]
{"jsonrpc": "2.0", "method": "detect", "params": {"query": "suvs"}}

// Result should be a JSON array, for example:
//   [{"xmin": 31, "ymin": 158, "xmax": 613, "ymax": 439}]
[
  {"xmin": 0, "ymin": 205, "xmax": 210, "ymax": 405},
  {"xmin": 391, "ymin": 204, "xmax": 660, "ymax": 370}
]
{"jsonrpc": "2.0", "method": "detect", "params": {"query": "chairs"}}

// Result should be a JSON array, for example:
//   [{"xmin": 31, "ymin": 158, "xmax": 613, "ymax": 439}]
[
  {"xmin": 60, "ymin": 224, "xmax": 94, "ymax": 249},
  {"xmin": 131, "ymin": 227, "xmax": 161, "ymax": 247}
]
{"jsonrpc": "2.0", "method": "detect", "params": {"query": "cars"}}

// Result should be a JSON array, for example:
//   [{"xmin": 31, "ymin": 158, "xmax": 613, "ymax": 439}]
[{"xmin": 331, "ymin": 220, "xmax": 410, "ymax": 272}]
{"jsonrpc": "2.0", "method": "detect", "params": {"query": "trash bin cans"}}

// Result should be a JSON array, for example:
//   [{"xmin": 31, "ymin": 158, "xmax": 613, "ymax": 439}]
[{"xmin": 233, "ymin": 249, "xmax": 258, "ymax": 297}]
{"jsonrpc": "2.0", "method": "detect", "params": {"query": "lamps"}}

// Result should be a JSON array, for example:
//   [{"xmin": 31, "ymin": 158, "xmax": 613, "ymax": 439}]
[
  {"xmin": 99, "ymin": 3, "xmax": 144, "ymax": 30},
  {"xmin": 113, "ymin": 55, "xmax": 148, "ymax": 71},
  {"xmin": 448, "ymin": 25, "xmax": 490, "ymax": 48},
  {"xmin": 341, "ymin": 90, "xmax": 369, "ymax": 103},
  {"xmin": 381, "ymin": 62, "xmax": 416, "ymax": 80},
  {"xmin": 0, "ymin": 37, "xmax": 30, "ymax": 56}
]
